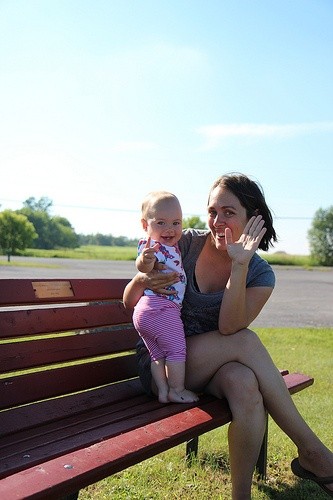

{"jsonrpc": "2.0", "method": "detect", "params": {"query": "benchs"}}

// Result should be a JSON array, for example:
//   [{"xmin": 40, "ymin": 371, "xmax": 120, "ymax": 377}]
[{"xmin": 0, "ymin": 271, "xmax": 315, "ymax": 500}]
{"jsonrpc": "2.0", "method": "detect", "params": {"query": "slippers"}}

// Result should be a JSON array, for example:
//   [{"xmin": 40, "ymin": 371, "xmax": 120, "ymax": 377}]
[{"xmin": 291, "ymin": 457, "xmax": 333, "ymax": 497}]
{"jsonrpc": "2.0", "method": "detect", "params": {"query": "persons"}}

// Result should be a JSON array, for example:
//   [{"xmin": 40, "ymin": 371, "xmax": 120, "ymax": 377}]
[
  {"xmin": 132, "ymin": 191, "xmax": 199, "ymax": 403},
  {"xmin": 122, "ymin": 172, "xmax": 332, "ymax": 500}
]
